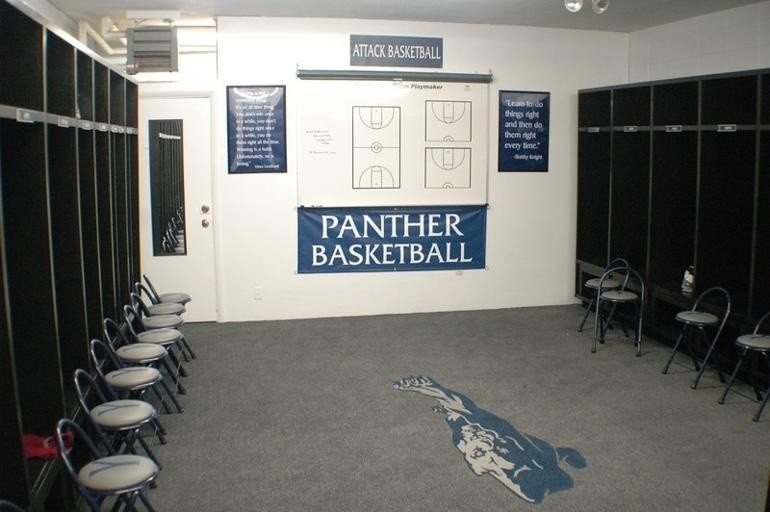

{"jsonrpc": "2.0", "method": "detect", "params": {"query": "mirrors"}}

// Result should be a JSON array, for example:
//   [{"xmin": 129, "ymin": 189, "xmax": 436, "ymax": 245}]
[{"xmin": 146, "ymin": 115, "xmax": 189, "ymax": 260}]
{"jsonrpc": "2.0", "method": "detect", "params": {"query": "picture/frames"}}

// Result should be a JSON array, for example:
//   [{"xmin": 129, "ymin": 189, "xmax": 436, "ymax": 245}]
[
  {"xmin": 225, "ymin": 84, "xmax": 289, "ymax": 175},
  {"xmin": 497, "ymin": 89, "xmax": 550, "ymax": 173}
]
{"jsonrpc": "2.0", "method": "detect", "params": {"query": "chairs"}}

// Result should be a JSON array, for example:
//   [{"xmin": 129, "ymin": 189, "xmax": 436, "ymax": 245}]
[
  {"xmin": 0, "ymin": 498, "xmax": 24, "ymax": 512},
  {"xmin": 571, "ymin": 257, "xmax": 631, "ymax": 344},
  {"xmin": 656, "ymin": 284, "xmax": 731, "ymax": 392},
  {"xmin": 714, "ymin": 306, "xmax": 769, "ymax": 424},
  {"xmin": 52, "ymin": 272, "xmax": 196, "ymax": 512},
  {"xmin": 589, "ymin": 267, "xmax": 647, "ymax": 361},
  {"xmin": 158, "ymin": 203, "xmax": 184, "ymax": 253}
]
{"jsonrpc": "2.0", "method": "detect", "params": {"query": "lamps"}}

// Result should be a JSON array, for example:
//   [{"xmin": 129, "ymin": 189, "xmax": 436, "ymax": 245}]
[
  {"xmin": 563, "ymin": 0, "xmax": 584, "ymax": 15},
  {"xmin": 591, "ymin": 0, "xmax": 611, "ymax": 15}
]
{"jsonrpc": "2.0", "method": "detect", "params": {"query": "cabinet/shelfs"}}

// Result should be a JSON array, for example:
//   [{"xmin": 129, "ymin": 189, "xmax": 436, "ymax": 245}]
[
  {"xmin": 575, "ymin": 67, "xmax": 768, "ymax": 389},
  {"xmin": 0, "ymin": 0, "xmax": 145, "ymax": 512},
  {"xmin": 147, "ymin": 121, "xmax": 185, "ymax": 254}
]
